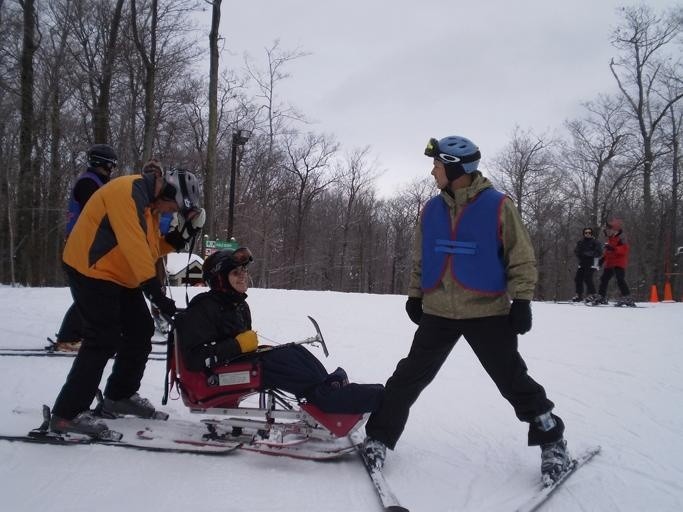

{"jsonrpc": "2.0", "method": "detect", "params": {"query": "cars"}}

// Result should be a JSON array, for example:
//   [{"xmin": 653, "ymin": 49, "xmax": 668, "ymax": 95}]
[{"xmin": 673, "ymin": 246, "xmax": 682, "ymax": 267}]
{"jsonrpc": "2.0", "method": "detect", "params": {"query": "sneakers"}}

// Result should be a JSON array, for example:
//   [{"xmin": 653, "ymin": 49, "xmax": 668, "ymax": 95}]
[
  {"xmin": 51, "ymin": 412, "xmax": 107, "ymax": 434},
  {"xmin": 540, "ymin": 440, "xmax": 570, "ymax": 476},
  {"xmin": 104, "ymin": 394, "xmax": 155, "ymax": 415},
  {"xmin": 57, "ymin": 341, "xmax": 81, "ymax": 352},
  {"xmin": 573, "ymin": 294, "xmax": 634, "ymax": 306},
  {"xmin": 364, "ymin": 436, "xmax": 386, "ymax": 461}
]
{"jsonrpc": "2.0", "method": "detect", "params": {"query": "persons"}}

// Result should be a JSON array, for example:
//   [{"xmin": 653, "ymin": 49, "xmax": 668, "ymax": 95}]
[
  {"xmin": 53, "ymin": 144, "xmax": 116, "ymax": 357},
  {"xmin": 363, "ymin": 134, "xmax": 573, "ymax": 479},
  {"xmin": 592, "ymin": 217, "xmax": 636, "ymax": 307},
  {"xmin": 140, "ymin": 158, "xmax": 175, "ymax": 333},
  {"xmin": 175, "ymin": 247, "xmax": 381, "ymax": 412},
  {"xmin": 575, "ymin": 227, "xmax": 602, "ymax": 302},
  {"xmin": 50, "ymin": 168, "xmax": 207, "ymax": 438}
]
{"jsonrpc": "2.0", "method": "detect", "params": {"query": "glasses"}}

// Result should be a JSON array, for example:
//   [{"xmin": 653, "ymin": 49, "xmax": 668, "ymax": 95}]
[
  {"xmin": 425, "ymin": 138, "xmax": 438, "ymax": 157},
  {"xmin": 183, "ymin": 206, "xmax": 202, "ymax": 221},
  {"xmin": 232, "ymin": 247, "xmax": 253, "ymax": 264}
]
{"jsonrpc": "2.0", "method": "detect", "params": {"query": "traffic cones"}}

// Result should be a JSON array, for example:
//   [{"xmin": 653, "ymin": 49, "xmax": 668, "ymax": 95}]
[
  {"xmin": 647, "ymin": 284, "xmax": 659, "ymax": 303},
  {"xmin": 660, "ymin": 282, "xmax": 676, "ymax": 303}
]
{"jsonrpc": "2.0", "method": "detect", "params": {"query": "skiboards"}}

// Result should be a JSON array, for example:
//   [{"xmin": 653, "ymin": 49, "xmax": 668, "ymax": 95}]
[
  {"xmin": 557, "ymin": 300, "xmax": 654, "ymax": 308},
  {"xmin": 0, "ymin": 347, "xmax": 169, "ymax": 362},
  {"xmin": 0, "ymin": 410, "xmax": 241, "ymax": 456},
  {"xmin": 349, "ymin": 429, "xmax": 601, "ymax": 511}
]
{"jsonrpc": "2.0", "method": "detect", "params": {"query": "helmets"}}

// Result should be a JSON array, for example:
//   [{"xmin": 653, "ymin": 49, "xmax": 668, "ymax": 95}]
[
  {"xmin": 202, "ymin": 249, "xmax": 248, "ymax": 287},
  {"xmin": 87, "ymin": 144, "xmax": 118, "ymax": 171},
  {"xmin": 163, "ymin": 170, "xmax": 199, "ymax": 214},
  {"xmin": 435, "ymin": 136, "xmax": 480, "ymax": 179}
]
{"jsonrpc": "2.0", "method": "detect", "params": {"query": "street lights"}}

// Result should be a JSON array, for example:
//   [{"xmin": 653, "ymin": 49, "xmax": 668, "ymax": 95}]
[{"xmin": 226, "ymin": 129, "xmax": 252, "ymax": 242}]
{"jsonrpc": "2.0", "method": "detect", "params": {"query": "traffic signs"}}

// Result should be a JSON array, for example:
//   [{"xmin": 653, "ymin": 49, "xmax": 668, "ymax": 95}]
[
  {"xmin": 205, "ymin": 248, "xmax": 218, "ymax": 256},
  {"xmin": 205, "ymin": 241, "xmax": 238, "ymax": 249}
]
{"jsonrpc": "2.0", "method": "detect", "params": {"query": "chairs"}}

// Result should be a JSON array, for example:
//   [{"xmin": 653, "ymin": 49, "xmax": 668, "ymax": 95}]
[{"xmin": 167, "ymin": 322, "xmax": 274, "ymax": 404}]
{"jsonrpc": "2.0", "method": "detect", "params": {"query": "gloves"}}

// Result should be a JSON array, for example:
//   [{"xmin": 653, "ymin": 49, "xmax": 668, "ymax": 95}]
[
  {"xmin": 406, "ymin": 300, "xmax": 422, "ymax": 324},
  {"xmin": 235, "ymin": 330, "xmax": 258, "ymax": 353},
  {"xmin": 510, "ymin": 302, "xmax": 531, "ymax": 334},
  {"xmin": 143, "ymin": 283, "xmax": 176, "ymax": 316}
]
